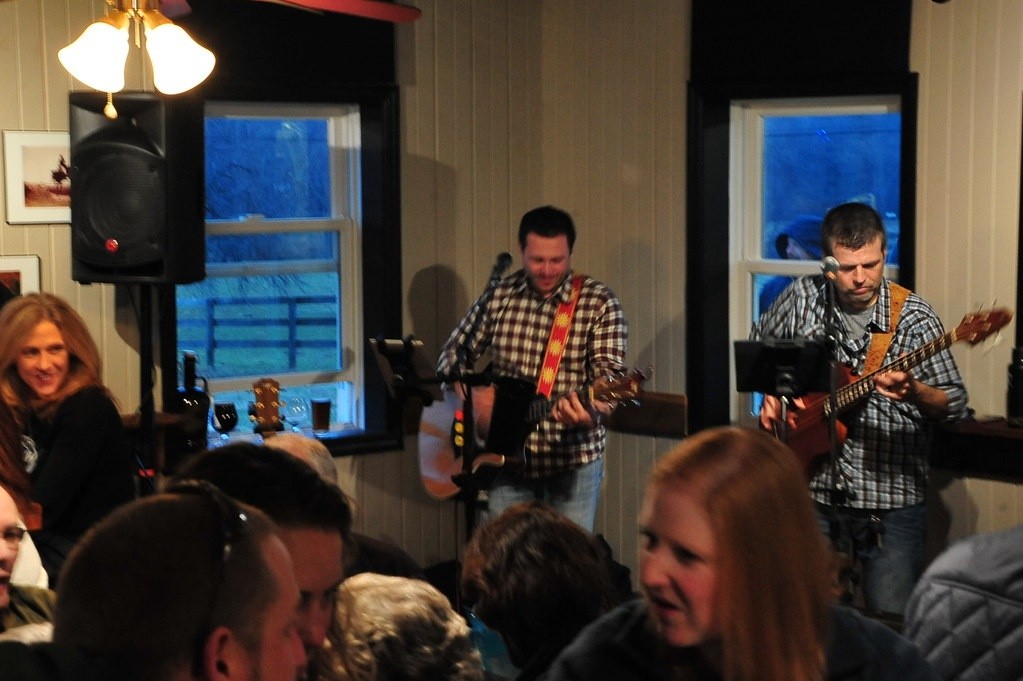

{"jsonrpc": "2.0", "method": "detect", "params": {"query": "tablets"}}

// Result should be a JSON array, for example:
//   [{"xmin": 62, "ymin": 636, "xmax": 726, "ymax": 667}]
[
  {"xmin": 371, "ymin": 339, "xmax": 445, "ymax": 404},
  {"xmin": 735, "ymin": 340, "xmax": 833, "ymax": 395}
]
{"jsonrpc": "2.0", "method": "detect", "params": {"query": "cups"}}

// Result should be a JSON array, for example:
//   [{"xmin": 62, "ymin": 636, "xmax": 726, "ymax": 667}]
[
  {"xmin": 210, "ymin": 401, "xmax": 238, "ymax": 433},
  {"xmin": 311, "ymin": 398, "xmax": 331, "ymax": 434}
]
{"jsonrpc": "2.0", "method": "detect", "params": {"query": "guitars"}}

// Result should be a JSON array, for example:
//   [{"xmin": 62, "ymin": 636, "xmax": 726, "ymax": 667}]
[
  {"xmin": 757, "ymin": 296, "xmax": 1015, "ymax": 475},
  {"xmin": 418, "ymin": 365, "xmax": 654, "ymax": 499},
  {"xmin": 247, "ymin": 375, "xmax": 287, "ymax": 439}
]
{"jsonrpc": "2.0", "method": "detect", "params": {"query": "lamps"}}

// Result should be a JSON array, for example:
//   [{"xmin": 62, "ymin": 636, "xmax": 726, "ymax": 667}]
[{"xmin": 57, "ymin": 0, "xmax": 217, "ymax": 118}]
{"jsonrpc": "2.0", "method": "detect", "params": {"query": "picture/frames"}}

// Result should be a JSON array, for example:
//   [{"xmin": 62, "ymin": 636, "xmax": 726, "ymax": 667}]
[
  {"xmin": 2, "ymin": 128, "xmax": 72, "ymax": 225},
  {"xmin": 0, "ymin": 254, "xmax": 41, "ymax": 309}
]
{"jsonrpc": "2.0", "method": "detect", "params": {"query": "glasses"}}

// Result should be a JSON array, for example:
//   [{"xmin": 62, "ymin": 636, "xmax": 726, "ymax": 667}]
[{"xmin": 165, "ymin": 480, "xmax": 245, "ymax": 681}]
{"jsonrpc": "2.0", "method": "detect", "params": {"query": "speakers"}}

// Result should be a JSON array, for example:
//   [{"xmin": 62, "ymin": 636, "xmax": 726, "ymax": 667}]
[{"xmin": 68, "ymin": 89, "xmax": 207, "ymax": 286}]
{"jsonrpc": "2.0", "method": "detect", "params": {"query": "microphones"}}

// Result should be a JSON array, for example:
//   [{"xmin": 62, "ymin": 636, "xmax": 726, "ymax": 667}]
[
  {"xmin": 820, "ymin": 256, "xmax": 840, "ymax": 281},
  {"xmin": 485, "ymin": 252, "xmax": 514, "ymax": 288}
]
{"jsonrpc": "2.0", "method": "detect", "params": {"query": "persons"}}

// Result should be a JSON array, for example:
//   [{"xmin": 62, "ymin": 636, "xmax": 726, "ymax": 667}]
[
  {"xmin": 0, "ymin": 290, "xmax": 135, "ymax": 588},
  {"xmin": 751, "ymin": 203, "xmax": 967, "ymax": 622},
  {"xmin": 434, "ymin": 205, "xmax": 628, "ymax": 535},
  {"xmin": 0, "ymin": 423, "xmax": 1023, "ymax": 681}
]
